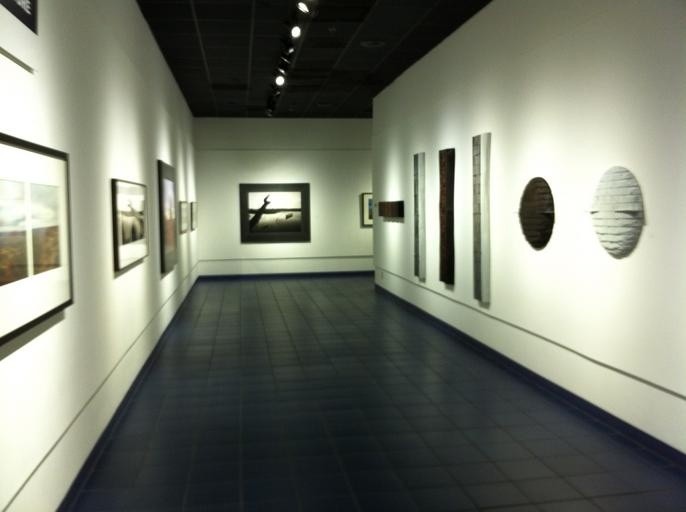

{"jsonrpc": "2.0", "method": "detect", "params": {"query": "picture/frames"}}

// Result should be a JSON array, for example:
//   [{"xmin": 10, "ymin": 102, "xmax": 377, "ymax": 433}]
[
  {"xmin": 361, "ymin": 193, "xmax": 375, "ymax": 228},
  {"xmin": 111, "ymin": 177, "xmax": 151, "ymax": 271},
  {"xmin": 179, "ymin": 200, "xmax": 199, "ymax": 234},
  {"xmin": 240, "ymin": 182, "xmax": 311, "ymax": 243},
  {"xmin": 0, "ymin": 131, "xmax": 74, "ymax": 346},
  {"xmin": 0, "ymin": 0, "xmax": 39, "ymax": 72},
  {"xmin": 156, "ymin": 159, "xmax": 179, "ymax": 275}
]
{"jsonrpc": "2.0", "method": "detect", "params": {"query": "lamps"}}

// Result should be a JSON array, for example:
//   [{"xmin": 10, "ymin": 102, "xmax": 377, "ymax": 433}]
[{"xmin": 273, "ymin": 2, "xmax": 314, "ymax": 88}]
